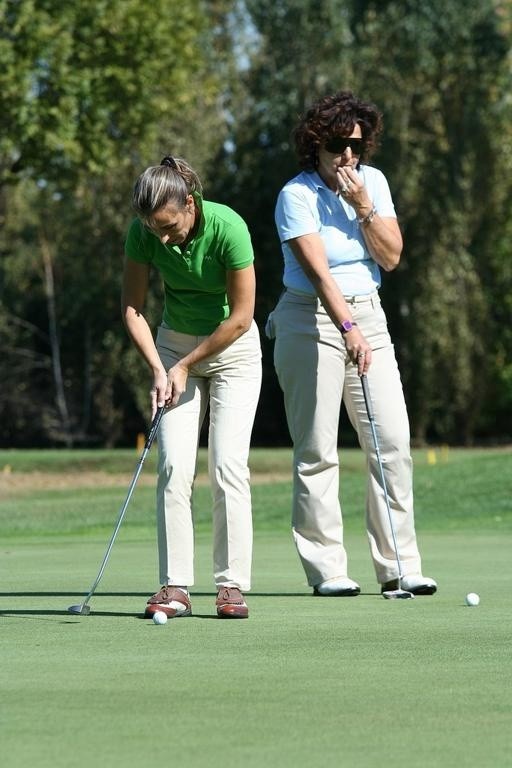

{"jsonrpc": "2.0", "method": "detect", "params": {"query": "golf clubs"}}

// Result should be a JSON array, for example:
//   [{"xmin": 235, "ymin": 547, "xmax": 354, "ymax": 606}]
[
  {"xmin": 356, "ymin": 363, "xmax": 413, "ymax": 600},
  {"xmin": 66, "ymin": 401, "xmax": 167, "ymax": 615}
]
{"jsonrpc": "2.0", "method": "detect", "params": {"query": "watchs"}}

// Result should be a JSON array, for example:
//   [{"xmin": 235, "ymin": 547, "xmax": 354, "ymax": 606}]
[{"xmin": 338, "ymin": 320, "xmax": 357, "ymax": 333}]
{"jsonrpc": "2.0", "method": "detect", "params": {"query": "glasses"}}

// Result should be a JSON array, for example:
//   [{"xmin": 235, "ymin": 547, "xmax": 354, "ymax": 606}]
[{"xmin": 324, "ymin": 130, "xmax": 368, "ymax": 155}]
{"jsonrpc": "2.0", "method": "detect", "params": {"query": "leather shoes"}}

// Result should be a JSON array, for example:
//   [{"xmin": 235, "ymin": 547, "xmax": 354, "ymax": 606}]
[
  {"xmin": 145, "ymin": 586, "xmax": 192, "ymax": 617},
  {"xmin": 381, "ymin": 575, "xmax": 437, "ymax": 595},
  {"xmin": 215, "ymin": 586, "xmax": 249, "ymax": 618},
  {"xmin": 313, "ymin": 577, "xmax": 361, "ymax": 596}
]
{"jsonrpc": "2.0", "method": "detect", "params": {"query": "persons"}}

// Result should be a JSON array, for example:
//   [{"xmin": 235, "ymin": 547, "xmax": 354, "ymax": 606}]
[
  {"xmin": 122, "ymin": 154, "xmax": 267, "ymax": 620},
  {"xmin": 267, "ymin": 88, "xmax": 438, "ymax": 595}
]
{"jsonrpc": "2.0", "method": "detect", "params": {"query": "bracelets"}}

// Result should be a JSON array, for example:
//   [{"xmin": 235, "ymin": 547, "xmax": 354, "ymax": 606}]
[{"xmin": 359, "ymin": 205, "xmax": 377, "ymax": 230}]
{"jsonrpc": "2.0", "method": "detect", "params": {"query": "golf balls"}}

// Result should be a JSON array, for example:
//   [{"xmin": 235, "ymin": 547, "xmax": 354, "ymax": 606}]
[
  {"xmin": 153, "ymin": 612, "xmax": 167, "ymax": 624},
  {"xmin": 465, "ymin": 592, "xmax": 479, "ymax": 604}
]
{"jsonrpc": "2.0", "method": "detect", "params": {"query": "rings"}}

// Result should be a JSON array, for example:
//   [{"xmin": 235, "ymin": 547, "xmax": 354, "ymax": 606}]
[
  {"xmin": 340, "ymin": 187, "xmax": 346, "ymax": 193},
  {"xmin": 357, "ymin": 351, "xmax": 366, "ymax": 358},
  {"xmin": 346, "ymin": 179, "xmax": 352, "ymax": 186}
]
